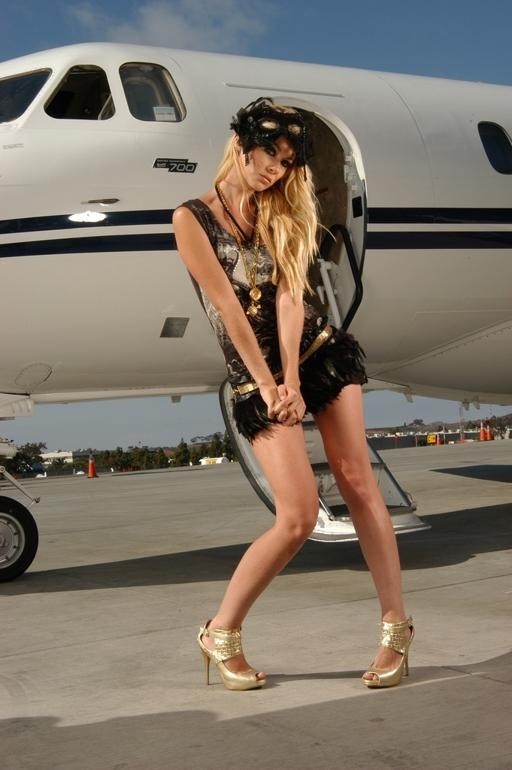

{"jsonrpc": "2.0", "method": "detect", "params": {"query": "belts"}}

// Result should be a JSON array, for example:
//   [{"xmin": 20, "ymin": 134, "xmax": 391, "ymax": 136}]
[{"xmin": 232, "ymin": 325, "xmax": 333, "ymax": 396}]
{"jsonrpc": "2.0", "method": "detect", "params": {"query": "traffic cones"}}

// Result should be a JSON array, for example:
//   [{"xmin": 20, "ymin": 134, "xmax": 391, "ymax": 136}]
[
  {"xmin": 480, "ymin": 419, "xmax": 493, "ymax": 441},
  {"xmin": 87, "ymin": 457, "xmax": 99, "ymax": 478}
]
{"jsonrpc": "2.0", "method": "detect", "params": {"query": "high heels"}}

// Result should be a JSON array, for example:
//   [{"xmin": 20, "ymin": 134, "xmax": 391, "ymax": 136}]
[
  {"xmin": 362, "ymin": 617, "xmax": 414, "ymax": 687},
  {"xmin": 197, "ymin": 620, "xmax": 266, "ymax": 690}
]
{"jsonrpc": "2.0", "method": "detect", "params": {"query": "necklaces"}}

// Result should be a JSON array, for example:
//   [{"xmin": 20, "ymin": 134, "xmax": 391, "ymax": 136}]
[{"xmin": 216, "ymin": 184, "xmax": 262, "ymax": 318}]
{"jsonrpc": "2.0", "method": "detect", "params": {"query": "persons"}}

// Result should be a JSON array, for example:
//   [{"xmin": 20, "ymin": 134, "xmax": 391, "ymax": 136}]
[{"xmin": 172, "ymin": 97, "xmax": 416, "ymax": 691}]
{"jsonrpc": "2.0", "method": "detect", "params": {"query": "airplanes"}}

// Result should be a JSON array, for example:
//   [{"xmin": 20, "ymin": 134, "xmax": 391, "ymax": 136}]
[{"xmin": 0, "ymin": 41, "xmax": 510, "ymax": 584}]
